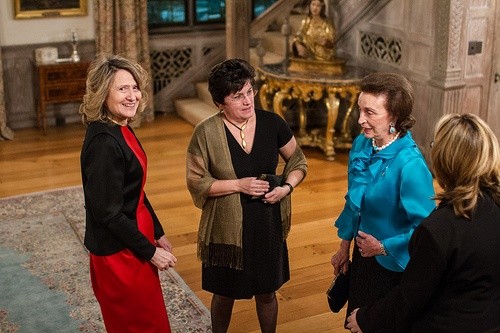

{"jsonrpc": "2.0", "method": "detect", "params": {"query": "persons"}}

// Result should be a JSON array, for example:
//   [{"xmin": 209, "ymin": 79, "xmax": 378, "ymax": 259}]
[
  {"xmin": 79, "ymin": 56, "xmax": 177, "ymax": 333},
  {"xmin": 293, "ymin": 0, "xmax": 335, "ymax": 62},
  {"xmin": 347, "ymin": 113, "xmax": 500, "ymax": 333},
  {"xmin": 186, "ymin": 59, "xmax": 307, "ymax": 333},
  {"xmin": 330, "ymin": 73, "xmax": 436, "ymax": 329}
]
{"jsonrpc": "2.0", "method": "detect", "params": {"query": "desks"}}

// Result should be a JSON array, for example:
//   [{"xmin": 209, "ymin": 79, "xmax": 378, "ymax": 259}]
[{"xmin": 257, "ymin": 63, "xmax": 372, "ymax": 162}]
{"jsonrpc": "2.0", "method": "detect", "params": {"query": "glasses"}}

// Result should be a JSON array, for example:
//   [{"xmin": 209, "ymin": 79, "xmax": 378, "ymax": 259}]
[
  {"xmin": 224, "ymin": 90, "xmax": 259, "ymax": 103},
  {"xmin": 430, "ymin": 141, "xmax": 436, "ymax": 149}
]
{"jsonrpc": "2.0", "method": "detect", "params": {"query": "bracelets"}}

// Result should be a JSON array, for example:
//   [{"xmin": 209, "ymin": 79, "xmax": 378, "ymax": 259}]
[
  {"xmin": 380, "ymin": 245, "xmax": 386, "ymax": 255},
  {"xmin": 282, "ymin": 183, "xmax": 293, "ymax": 195}
]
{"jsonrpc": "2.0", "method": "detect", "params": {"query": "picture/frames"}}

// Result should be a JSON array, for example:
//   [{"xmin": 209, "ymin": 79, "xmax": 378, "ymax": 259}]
[{"xmin": 14, "ymin": 0, "xmax": 88, "ymax": 19}]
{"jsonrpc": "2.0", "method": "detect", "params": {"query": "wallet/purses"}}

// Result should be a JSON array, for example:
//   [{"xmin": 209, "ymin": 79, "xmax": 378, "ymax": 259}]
[
  {"xmin": 251, "ymin": 174, "xmax": 285, "ymax": 199},
  {"xmin": 326, "ymin": 259, "xmax": 353, "ymax": 313}
]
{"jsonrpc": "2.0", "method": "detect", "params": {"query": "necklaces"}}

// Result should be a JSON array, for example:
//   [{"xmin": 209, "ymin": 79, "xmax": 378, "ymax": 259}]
[
  {"xmin": 224, "ymin": 114, "xmax": 248, "ymax": 148},
  {"xmin": 372, "ymin": 132, "xmax": 400, "ymax": 150}
]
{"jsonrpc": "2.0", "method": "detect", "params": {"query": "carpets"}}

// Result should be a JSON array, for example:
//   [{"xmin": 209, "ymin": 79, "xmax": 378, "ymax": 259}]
[{"xmin": 0, "ymin": 186, "xmax": 214, "ymax": 333}]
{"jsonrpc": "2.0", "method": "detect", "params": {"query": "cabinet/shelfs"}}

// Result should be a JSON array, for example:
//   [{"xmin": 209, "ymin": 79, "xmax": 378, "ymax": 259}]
[{"xmin": 33, "ymin": 61, "xmax": 92, "ymax": 130}]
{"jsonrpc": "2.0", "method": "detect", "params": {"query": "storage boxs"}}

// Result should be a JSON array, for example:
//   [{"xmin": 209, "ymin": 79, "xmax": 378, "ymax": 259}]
[{"xmin": 36, "ymin": 47, "xmax": 59, "ymax": 62}]
